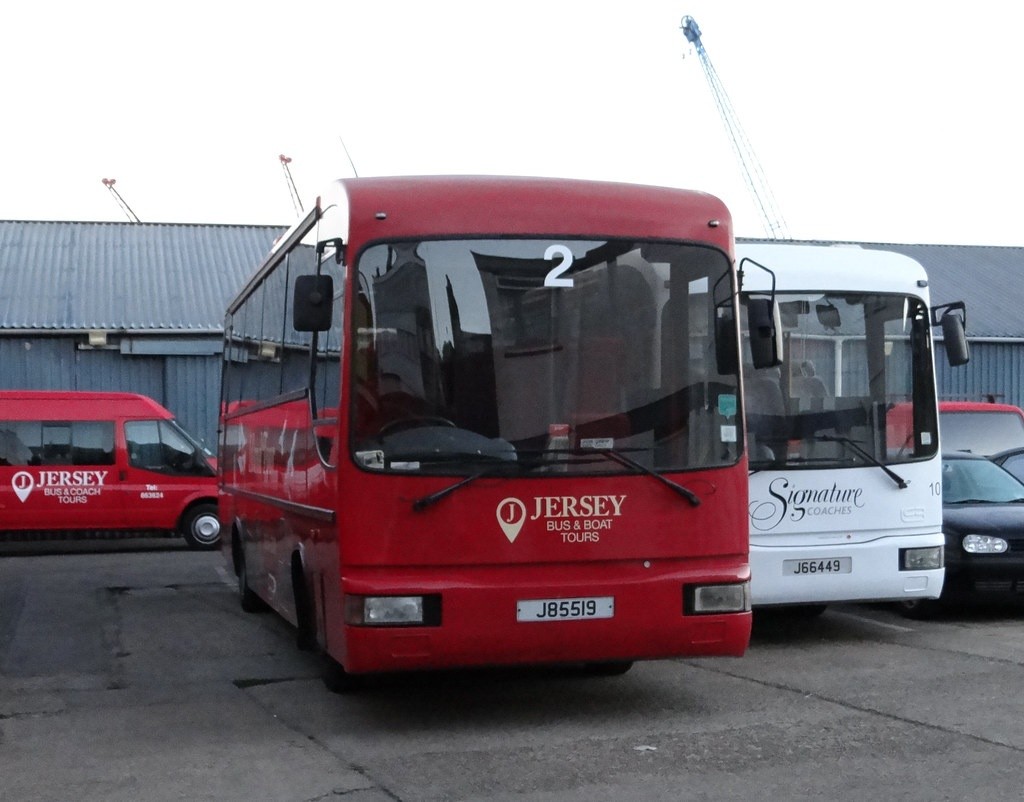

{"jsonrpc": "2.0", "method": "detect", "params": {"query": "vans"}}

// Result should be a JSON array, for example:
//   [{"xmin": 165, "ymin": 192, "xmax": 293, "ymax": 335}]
[{"xmin": 0, "ymin": 391, "xmax": 222, "ymax": 550}]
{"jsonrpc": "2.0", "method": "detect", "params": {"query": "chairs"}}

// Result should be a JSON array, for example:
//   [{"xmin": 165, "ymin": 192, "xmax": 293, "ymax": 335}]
[{"xmin": 742, "ymin": 358, "xmax": 829, "ymax": 415}]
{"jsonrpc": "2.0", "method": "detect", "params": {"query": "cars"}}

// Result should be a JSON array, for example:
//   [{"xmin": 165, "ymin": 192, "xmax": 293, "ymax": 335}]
[
  {"xmin": 974, "ymin": 447, "xmax": 1024, "ymax": 501},
  {"xmin": 887, "ymin": 448, "xmax": 1024, "ymax": 618},
  {"xmin": 886, "ymin": 401, "xmax": 1024, "ymax": 456}
]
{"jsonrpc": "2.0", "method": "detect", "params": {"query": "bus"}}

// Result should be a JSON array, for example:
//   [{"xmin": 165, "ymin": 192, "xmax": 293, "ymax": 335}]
[
  {"xmin": 217, "ymin": 173, "xmax": 752, "ymax": 691},
  {"xmin": 654, "ymin": 244, "xmax": 970, "ymax": 619}
]
